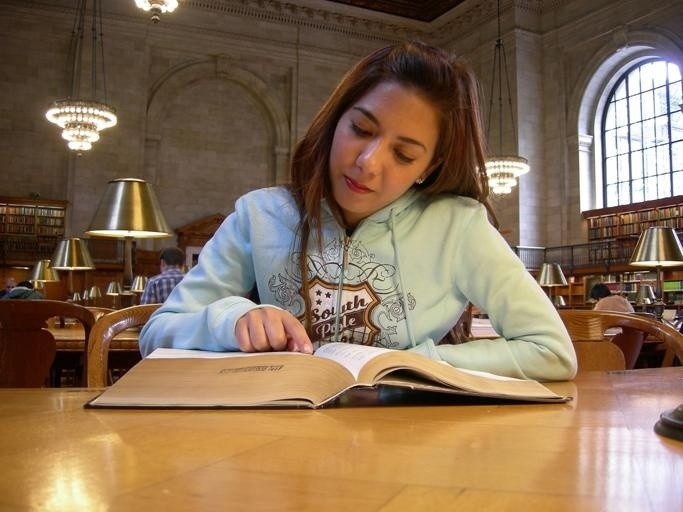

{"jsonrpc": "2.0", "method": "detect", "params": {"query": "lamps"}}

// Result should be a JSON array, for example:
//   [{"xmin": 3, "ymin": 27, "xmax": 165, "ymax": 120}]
[
  {"xmin": 26, "ymin": 235, "xmax": 151, "ymax": 312},
  {"xmin": 43, "ymin": 0, "xmax": 119, "ymax": 158},
  {"xmin": 82, "ymin": 177, "xmax": 174, "ymax": 331},
  {"xmin": 134, "ymin": 0, "xmax": 179, "ymax": 24},
  {"xmin": 474, "ymin": 0, "xmax": 530, "ymax": 196}
]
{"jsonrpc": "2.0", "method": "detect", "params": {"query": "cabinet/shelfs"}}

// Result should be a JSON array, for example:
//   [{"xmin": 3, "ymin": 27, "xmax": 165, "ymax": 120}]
[
  {"xmin": 1, "ymin": 197, "xmax": 65, "ymax": 264},
  {"xmin": 527, "ymin": 262, "xmax": 683, "ymax": 312},
  {"xmin": 581, "ymin": 192, "xmax": 683, "ymax": 265}
]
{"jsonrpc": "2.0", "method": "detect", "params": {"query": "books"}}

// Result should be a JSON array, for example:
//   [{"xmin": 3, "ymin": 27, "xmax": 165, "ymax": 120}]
[
  {"xmin": 1, "ymin": 201, "xmax": 65, "ymax": 255},
  {"xmin": 86, "ymin": 338, "xmax": 576, "ymax": 412},
  {"xmin": 585, "ymin": 203, "xmax": 683, "ymax": 316}
]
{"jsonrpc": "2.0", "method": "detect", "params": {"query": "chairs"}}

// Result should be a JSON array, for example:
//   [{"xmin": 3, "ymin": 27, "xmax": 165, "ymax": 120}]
[
  {"xmin": 0, "ymin": 291, "xmax": 165, "ymax": 390},
  {"xmin": 545, "ymin": 307, "xmax": 683, "ymax": 374}
]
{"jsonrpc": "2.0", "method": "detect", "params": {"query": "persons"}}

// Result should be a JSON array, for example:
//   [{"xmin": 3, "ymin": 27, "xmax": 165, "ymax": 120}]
[
  {"xmin": 1, "ymin": 279, "xmax": 42, "ymax": 301},
  {"xmin": 589, "ymin": 281, "xmax": 637, "ymax": 317},
  {"xmin": 139, "ymin": 247, "xmax": 182, "ymax": 306},
  {"xmin": 138, "ymin": 41, "xmax": 579, "ymax": 386}
]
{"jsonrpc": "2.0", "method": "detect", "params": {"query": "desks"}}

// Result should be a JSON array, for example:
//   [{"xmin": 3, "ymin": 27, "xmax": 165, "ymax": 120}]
[{"xmin": 1, "ymin": 364, "xmax": 683, "ymax": 512}]
